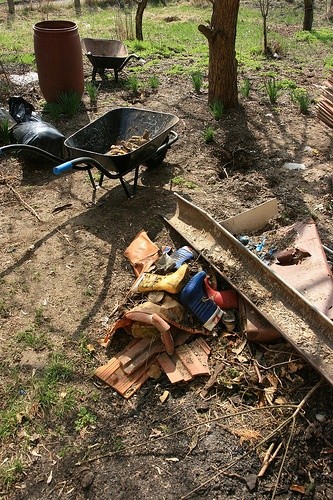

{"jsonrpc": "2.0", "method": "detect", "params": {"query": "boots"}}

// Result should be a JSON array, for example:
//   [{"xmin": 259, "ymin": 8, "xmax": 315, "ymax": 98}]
[
  {"xmin": 169, "ymin": 246, "xmax": 199, "ymax": 270},
  {"xmin": 181, "ymin": 270, "xmax": 225, "ymax": 332},
  {"xmin": 124, "ymin": 229, "xmax": 163, "ymax": 293},
  {"xmin": 204, "ymin": 273, "xmax": 238, "ymax": 309},
  {"xmin": 132, "ymin": 264, "xmax": 189, "ymax": 294}
]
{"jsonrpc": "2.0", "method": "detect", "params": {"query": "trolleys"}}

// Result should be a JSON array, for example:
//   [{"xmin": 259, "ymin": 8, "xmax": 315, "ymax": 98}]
[
  {"xmin": 0, "ymin": 106, "xmax": 180, "ymax": 201},
  {"xmin": 81, "ymin": 37, "xmax": 140, "ymax": 83}
]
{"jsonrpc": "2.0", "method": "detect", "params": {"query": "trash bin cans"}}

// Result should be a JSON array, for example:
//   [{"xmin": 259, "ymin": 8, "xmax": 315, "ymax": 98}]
[{"xmin": 33, "ymin": 19, "xmax": 84, "ymax": 105}]
